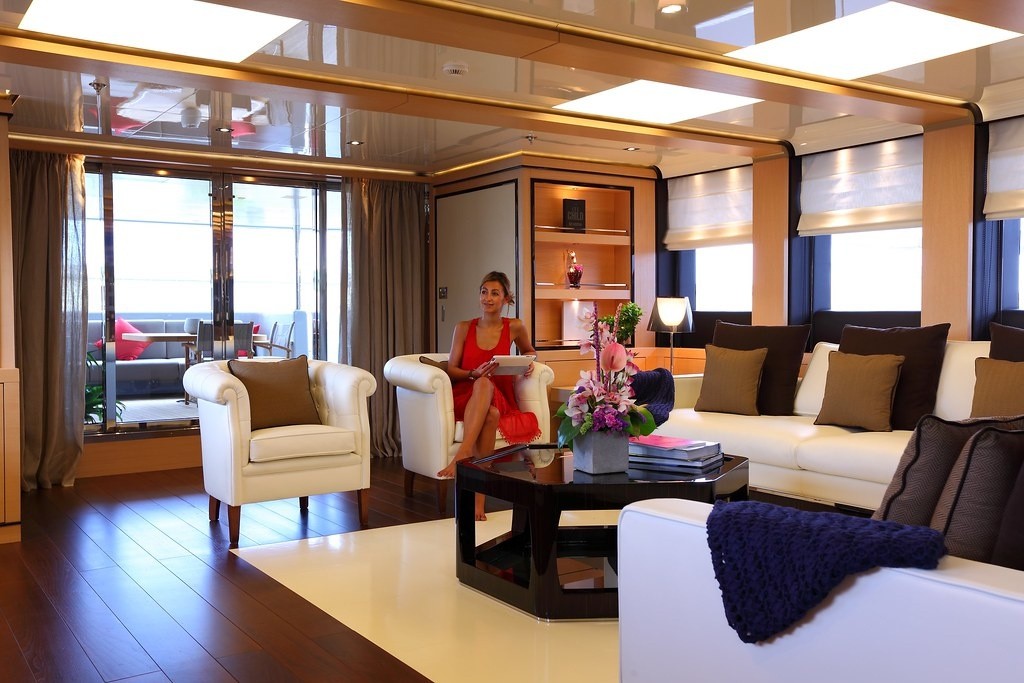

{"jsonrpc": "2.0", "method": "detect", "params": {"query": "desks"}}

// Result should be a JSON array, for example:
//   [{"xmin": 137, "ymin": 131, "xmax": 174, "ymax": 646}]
[{"xmin": 122, "ymin": 332, "xmax": 268, "ymax": 407}]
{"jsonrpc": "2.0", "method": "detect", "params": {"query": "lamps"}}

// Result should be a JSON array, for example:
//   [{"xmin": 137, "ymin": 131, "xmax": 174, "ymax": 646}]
[{"xmin": 647, "ymin": 296, "xmax": 696, "ymax": 378}]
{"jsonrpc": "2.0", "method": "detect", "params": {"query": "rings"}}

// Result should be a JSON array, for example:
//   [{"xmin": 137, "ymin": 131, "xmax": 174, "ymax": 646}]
[{"xmin": 488, "ymin": 373, "xmax": 491, "ymax": 377}]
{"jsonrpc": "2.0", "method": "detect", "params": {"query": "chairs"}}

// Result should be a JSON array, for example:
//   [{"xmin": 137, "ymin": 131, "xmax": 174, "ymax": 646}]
[
  {"xmin": 383, "ymin": 354, "xmax": 556, "ymax": 513},
  {"xmin": 181, "ymin": 320, "xmax": 294, "ymax": 405},
  {"xmin": 183, "ymin": 358, "xmax": 377, "ymax": 543}
]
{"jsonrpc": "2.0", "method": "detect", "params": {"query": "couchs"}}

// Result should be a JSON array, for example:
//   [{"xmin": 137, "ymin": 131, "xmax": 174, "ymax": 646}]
[
  {"xmin": 617, "ymin": 342, "xmax": 1024, "ymax": 683},
  {"xmin": 64, "ymin": 319, "xmax": 247, "ymax": 397}
]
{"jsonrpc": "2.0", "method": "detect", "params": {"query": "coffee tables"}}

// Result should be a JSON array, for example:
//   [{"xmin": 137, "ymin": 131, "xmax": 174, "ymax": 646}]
[{"xmin": 455, "ymin": 443, "xmax": 750, "ymax": 622}]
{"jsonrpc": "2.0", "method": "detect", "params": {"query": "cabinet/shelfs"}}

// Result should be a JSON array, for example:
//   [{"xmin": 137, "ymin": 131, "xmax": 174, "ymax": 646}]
[{"xmin": 524, "ymin": 155, "xmax": 658, "ymax": 351}]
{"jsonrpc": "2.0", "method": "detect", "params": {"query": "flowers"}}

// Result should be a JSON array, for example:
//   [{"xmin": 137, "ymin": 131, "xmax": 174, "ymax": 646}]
[{"xmin": 553, "ymin": 300, "xmax": 656, "ymax": 453}]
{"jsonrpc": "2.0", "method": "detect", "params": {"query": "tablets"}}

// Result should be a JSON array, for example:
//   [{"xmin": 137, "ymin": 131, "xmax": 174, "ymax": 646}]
[{"xmin": 489, "ymin": 354, "xmax": 536, "ymax": 375}]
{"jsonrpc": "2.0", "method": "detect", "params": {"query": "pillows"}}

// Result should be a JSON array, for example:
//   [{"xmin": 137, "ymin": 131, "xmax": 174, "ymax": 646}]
[
  {"xmin": 695, "ymin": 320, "xmax": 1024, "ymax": 574},
  {"xmin": 227, "ymin": 354, "xmax": 322, "ymax": 434},
  {"xmin": 94, "ymin": 317, "xmax": 153, "ymax": 361}
]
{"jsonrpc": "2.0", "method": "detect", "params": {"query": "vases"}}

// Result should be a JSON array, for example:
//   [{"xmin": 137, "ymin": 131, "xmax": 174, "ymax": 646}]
[{"xmin": 573, "ymin": 430, "xmax": 629, "ymax": 475}]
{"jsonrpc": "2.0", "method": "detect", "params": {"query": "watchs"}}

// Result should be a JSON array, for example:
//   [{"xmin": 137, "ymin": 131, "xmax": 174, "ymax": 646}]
[{"xmin": 468, "ymin": 369, "xmax": 478, "ymax": 380}]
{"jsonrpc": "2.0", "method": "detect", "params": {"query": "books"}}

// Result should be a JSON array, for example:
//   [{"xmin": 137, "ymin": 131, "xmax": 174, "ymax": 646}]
[{"xmin": 628, "ymin": 433, "xmax": 724, "ymax": 466}]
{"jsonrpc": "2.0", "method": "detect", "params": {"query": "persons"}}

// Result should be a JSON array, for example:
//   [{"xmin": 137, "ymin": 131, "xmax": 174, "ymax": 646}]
[{"xmin": 437, "ymin": 271, "xmax": 538, "ymax": 521}]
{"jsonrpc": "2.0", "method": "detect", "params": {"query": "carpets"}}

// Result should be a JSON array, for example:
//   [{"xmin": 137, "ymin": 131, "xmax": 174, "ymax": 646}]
[{"xmin": 229, "ymin": 507, "xmax": 624, "ymax": 683}]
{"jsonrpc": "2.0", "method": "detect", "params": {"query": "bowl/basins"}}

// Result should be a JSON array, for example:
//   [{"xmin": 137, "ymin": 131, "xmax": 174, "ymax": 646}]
[
  {"xmin": 253, "ymin": 334, "xmax": 267, "ymax": 340},
  {"xmin": 183, "ymin": 318, "xmax": 199, "ymax": 334}
]
